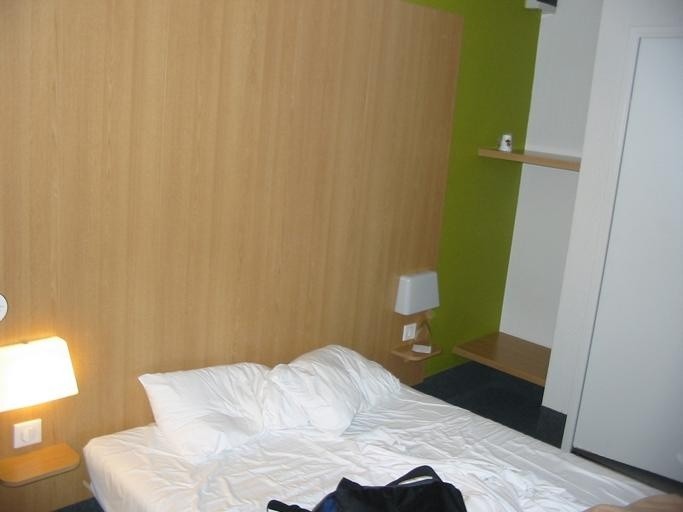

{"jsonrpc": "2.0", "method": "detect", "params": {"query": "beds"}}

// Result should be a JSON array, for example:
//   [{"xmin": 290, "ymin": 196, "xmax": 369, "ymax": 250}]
[{"xmin": 82, "ymin": 352, "xmax": 683, "ymax": 512}]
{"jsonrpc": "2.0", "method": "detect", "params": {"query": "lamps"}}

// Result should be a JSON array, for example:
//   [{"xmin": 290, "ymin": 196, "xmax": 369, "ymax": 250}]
[{"xmin": 395, "ymin": 270, "xmax": 439, "ymax": 317}]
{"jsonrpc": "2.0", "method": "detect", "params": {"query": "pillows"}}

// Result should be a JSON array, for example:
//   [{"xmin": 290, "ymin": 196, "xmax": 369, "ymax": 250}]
[{"xmin": 137, "ymin": 343, "xmax": 398, "ymax": 469}]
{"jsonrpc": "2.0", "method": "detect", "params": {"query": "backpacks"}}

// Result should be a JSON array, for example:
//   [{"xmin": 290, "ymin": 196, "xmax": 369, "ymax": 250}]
[{"xmin": 266, "ymin": 465, "xmax": 468, "ymax": 512}]
